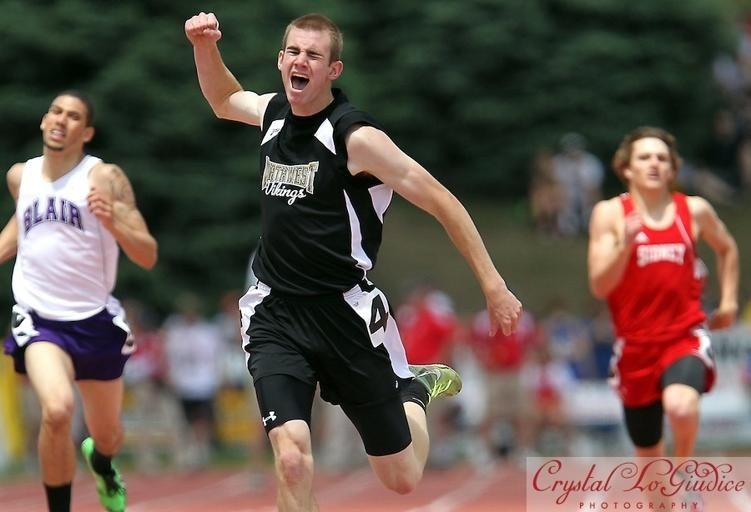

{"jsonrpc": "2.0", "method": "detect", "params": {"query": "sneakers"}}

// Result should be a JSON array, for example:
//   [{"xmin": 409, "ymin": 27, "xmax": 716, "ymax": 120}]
[
  {"xmin": 408, "ymin": 363, "xmax": 463, "ymax": 406},
  {"xmin": 80, "ymin": 435, "xmax": 127, "ymax": 512}
]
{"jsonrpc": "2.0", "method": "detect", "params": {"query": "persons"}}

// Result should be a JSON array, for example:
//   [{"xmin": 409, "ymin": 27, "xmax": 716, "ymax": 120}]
[
  {"xmin": 533, "ymin": 131, "xmax": 606, "ymax": 238},
  {"xmin": 586, "ymin": 125, "xmax": 740, "ymax": 512},
  {"xmin": 637, "ymin": 100, "xmax": 751, "ymax": 201},
  {"xmin": 0, "ymin": 286, "xmax": 625, "ymax": 498},
  {"xmin": 176, "ymin": 11, "xmax": 523, "ymax": 512},
  {"xmin": 0, "ymin": 88, "xmax": 156, "ymax": 512}
]
